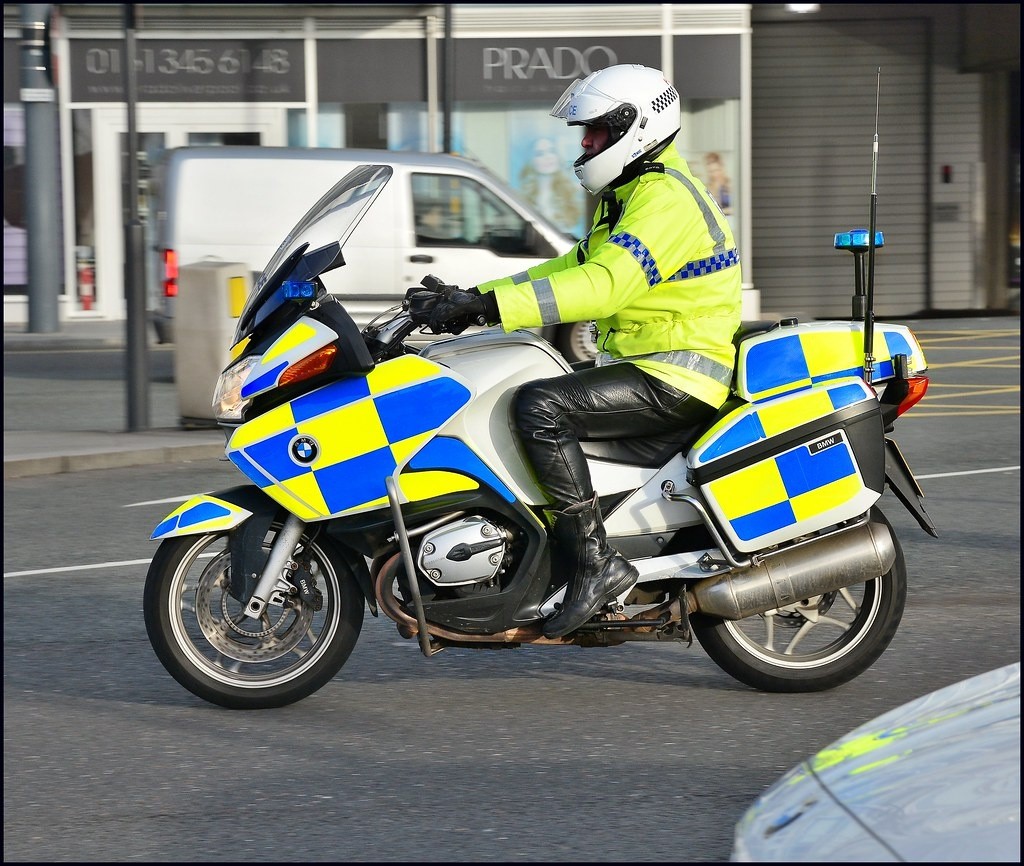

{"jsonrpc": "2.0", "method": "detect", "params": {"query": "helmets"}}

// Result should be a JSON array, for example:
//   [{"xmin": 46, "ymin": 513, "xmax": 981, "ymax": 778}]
[{"xmin": 554, "ymin": 68, "xmax": 681, "ymax": 196}]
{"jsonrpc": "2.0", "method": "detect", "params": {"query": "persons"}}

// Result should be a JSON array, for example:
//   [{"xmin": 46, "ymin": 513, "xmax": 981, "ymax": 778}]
[
  {"xmin": 412, "ymin": 193, "xmax": 464, "ymax": 244},
  {"xmin": 703, "ymin": 152, "xmax": 731, "ymax": 217},
  {"xmin": 520, "ymin": 138, "xmax": 579, "ymax": 232},
  {"xmin": 399, "ymin": 64, "xmax": 743, "ymax": 641}
]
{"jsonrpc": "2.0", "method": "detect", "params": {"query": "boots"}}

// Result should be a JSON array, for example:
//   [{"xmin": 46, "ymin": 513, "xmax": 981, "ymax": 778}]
[{"xmin": 537, "ymin": 493, "xmax": 637, "ymax": 642}]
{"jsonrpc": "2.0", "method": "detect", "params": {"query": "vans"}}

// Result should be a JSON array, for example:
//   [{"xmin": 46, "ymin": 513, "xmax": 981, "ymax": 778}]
[{"xmin": 149, "ymin": 145, "xmax": 585, "ymax": 348}]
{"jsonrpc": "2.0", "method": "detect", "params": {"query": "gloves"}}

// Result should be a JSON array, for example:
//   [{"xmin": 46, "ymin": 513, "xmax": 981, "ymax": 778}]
[{"xmin": 428, "ymin": 288, "xmax": 489, "ymax": 334}]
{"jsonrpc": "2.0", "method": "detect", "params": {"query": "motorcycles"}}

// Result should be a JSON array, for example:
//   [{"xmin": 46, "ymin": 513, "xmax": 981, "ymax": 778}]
[{"xmin": 138, "ymin": 58, "xmax": 937, "ymax": 712}]
